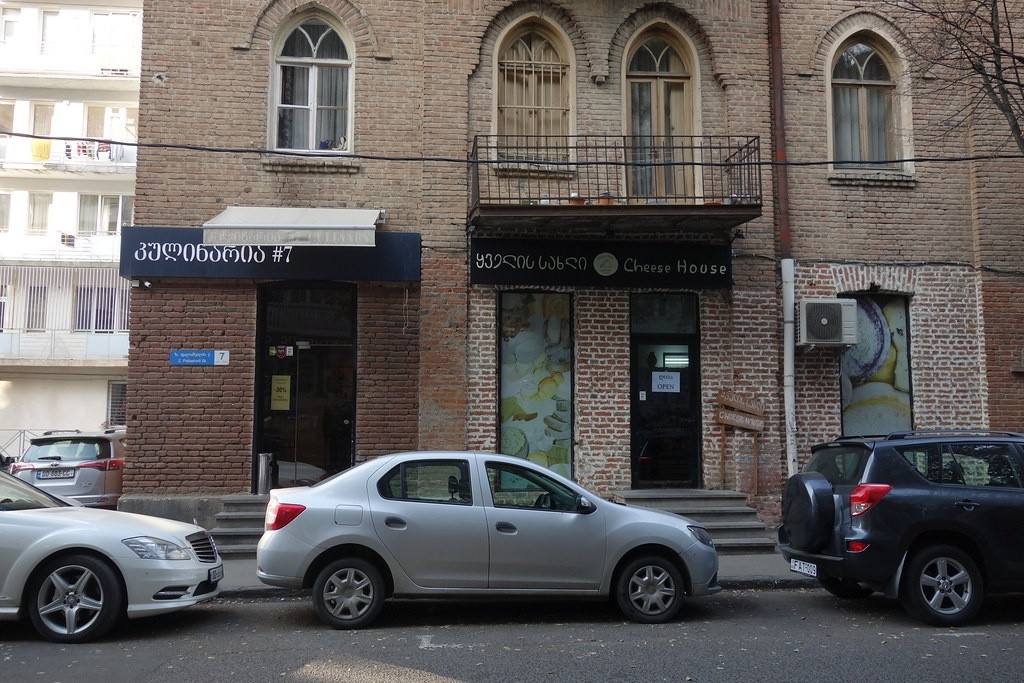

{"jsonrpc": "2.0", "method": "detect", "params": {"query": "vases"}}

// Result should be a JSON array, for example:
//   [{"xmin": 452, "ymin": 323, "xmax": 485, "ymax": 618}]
[
  {"xmin": 568, "ymin": 193, "xmax": 586, "ymax": 205},
  {"xmin": 596, "ymin": 191, "xmax": 616, "ymax": 206},
  {"xmin": 704, "ymin": 201, "xmax": 721, "ymax": 205}
]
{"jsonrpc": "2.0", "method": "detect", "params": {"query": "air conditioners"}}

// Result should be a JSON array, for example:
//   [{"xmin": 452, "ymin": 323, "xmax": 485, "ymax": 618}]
[
  {"xmin": 60, "ymin": 232, "xmax": 75, "ymax": 247},
  {"xmin": 793, "ymin": 298, "xmax": 858, "ymax": 358}
]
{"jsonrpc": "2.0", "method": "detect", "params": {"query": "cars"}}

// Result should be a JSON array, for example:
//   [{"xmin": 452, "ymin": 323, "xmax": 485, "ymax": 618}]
[
  {"xmin": 6, "ymin": 428, "xmax": 125, "ymax": 510},
  {"xmin": 254, "ymin": 451, "xmax": 719, "ymax": 625},
  {"xmin": 776, "ymin": 429, "xmax": 1024, "ymax": 629},
  {"xmin": 0, "ymin": 470, "xmax": 225, "ymax": 644}
]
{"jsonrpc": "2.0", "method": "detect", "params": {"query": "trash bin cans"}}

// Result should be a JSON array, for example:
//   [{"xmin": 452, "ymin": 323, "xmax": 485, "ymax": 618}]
[{"xmin": 257, "ymin": 453, "xmax": 273, "ymax": 494}]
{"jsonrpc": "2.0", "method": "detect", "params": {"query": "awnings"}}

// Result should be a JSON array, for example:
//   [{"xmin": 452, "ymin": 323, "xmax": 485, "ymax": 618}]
[{"xmin": 201, "ymin": 206, "xmax": 386, "ymax": 247}]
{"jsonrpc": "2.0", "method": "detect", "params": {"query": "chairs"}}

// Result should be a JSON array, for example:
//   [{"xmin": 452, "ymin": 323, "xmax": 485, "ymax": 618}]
[
  {"xmin": 983, "ymin": 455, "xmax": 1012, "ymax": 488},
  {"xmin": 942, "ymin": 460, "xmax": 966, "ymax": 485},
  {"xmin": 447, "ymin": 475, "xmax": 470, "ymax": 504},
  {"xmin": 78, "ymin": 444, "xmax": 99, "ymax": 460}
]
